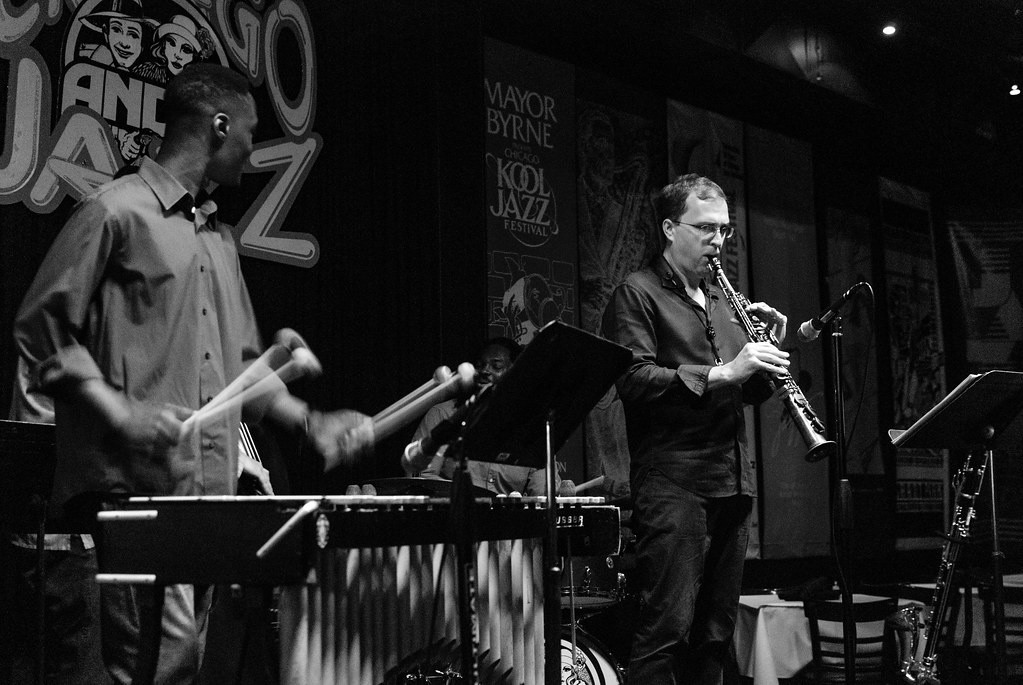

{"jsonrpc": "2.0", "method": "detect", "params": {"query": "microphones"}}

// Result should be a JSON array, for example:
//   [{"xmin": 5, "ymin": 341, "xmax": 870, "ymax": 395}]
[{"xmin": 797, "ymin": 282, "xmax": 865, "ymax": 343}]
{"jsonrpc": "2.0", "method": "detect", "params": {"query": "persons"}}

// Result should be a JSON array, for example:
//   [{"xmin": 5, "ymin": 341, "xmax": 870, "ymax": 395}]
[
  {"xmin": 601, "ymin": 172, "xmax": 791, "ymax": 685},
  {"xmin": 402, "ymin": 337, "xmax": 561, "ymax": 497},
  {"xmin": 10, "ymin": 62, "xmax": 376, "ymax": 684}
]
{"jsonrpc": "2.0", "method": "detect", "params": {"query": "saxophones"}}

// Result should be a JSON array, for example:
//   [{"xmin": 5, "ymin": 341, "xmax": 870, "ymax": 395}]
[{"xmin": 886, "ymin": 445, "xmax": 990, "ymax": 684}]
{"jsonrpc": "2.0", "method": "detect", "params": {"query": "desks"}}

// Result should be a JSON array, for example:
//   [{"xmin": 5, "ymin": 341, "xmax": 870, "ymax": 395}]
[
  {"xmin": 729, "ymin": 592, "xmax": 925, "ymax": 685},
  {"xmin": 912, "ymin": 573, "xmax": 1023, "ymax": 648}
]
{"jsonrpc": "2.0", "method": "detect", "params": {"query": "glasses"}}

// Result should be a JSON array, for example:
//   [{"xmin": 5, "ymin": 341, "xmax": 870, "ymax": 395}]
[{"xmin": 672, "ymin": 221, "xmax": 734, "ymax": 238}]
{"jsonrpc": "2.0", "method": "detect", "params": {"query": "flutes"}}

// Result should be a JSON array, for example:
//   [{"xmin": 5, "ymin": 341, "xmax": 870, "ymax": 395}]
[{"xmin": 706, "ymin": 255, "xmax": 839, "ymax": 464}]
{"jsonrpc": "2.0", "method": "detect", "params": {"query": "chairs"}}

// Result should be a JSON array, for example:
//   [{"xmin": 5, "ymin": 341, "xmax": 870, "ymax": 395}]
[
  {"xmin": 804, "ymin": 597, "xmax": 898, "ymax": 685},
  {"xmin": 913, "ymin": 588, "xmax": 962, "ymax": 658},
  {"xmin": 978, "ymin": 582, "xmax": 1023, "ymax": 685}
]
